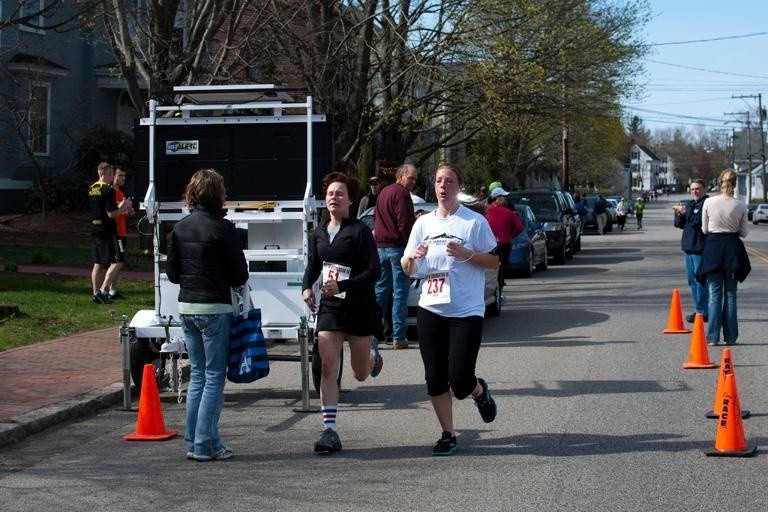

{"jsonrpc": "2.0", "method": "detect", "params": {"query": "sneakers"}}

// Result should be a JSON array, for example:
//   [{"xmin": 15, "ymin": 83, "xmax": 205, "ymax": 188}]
[
  {"xmin": 431, "ymin": 430, "xmax": 458, "ymax": 457},
  {"xmin": 313, "ymin": 427, "xmax": 343, "ymax": 453},
  {"xmin": 686, "ymin": 311, "xmax": 709, "ymax": 323},
  {"xmin": 392, "ymin": 337, "xmax": 410, "ymax": 350},
  {"xmin": 107, "ymin": 291, "xmax": 129, "ymax": 300},
  {"xmin": 187, "ymin": 444, "xmax": 235, "ymax": 461},
  {"xmin": 369, "ymin": 335, "xmax": 384, "ymax": 378},
  {"xmin": 92, "ymin": 290, "xmax": 112, "ymax": 306},
  {"xmin": 472, "ymin": 376, "xmax": 498, "ymax": 424}
]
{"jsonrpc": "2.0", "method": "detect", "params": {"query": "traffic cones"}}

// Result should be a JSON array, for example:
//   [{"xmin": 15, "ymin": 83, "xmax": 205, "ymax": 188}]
[
  {"xmin": 663, "ymin": 289, "xmax": 757, "ymax": 461},
  {"xmin": 125, "ymin": 362, "xmax": 176, "ymax": 443}
]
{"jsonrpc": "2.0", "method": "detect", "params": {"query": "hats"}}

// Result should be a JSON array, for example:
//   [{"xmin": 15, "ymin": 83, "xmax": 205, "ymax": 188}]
[
  {"xmin": 369, "ymin": 176, "xmax": 379, "ymax": 181},
  {"xmin": 490, "ymin": 186, "xmax": 510, "ymax": 199},
  {"xmin": 488, "ymin": 181, "xmax": 504, "ymax": 191}
]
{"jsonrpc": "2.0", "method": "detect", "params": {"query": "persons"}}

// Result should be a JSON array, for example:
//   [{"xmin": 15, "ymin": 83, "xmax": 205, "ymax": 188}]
[
  {"xmin": 486, "ymin": 187, "xmax": 510, "ymax": 209},
  {"xmin": 87, "ymin": 161, "xmax": 134, "ymax": 304},
  {"xmin": 163, "ymin": 168, "xmax": 250, "ymax": 463},
  {"xmin": 398, "ymin": 162, "xmax": 502, "ymax": 456},
  {"xmin": 370, "ymin": 163, "xmax": 424, "ymax": 351},
  {"xmin": 568, "ymin": 183, "xmax": 670, "ymax": 234},
  {"xmin": 103, "ymin": 164, "xmax": 133, "ymax": 300},
  {"xmin": 700, "ymin": 168, "xmax": 752, "ymax": 347},
  {"xmin": 358, "ymin": 174, "xmax": 382, "ymax": 220},
  {"xmin": 299, "ymin": 170, "xmax": 384, "ymax": 454},
  {"xmin": 476, "ymin": 185, "xmax": 488, "ymax": 201},
  {"xmin": 408, "ymin": 182, "xmax": 426, "ymax": 204},
  {"xmin": 671, "ymin": 178, "xmax": 710, "ymax": 323},
  {"xmin": 484, "ymin": 197, "xmax": 524, "ymax": 306}
]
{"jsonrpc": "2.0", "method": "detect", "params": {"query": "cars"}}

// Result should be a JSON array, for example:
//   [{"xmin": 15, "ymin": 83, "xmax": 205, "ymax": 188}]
[
  {"xmin": 630, "ymin": 185, "xmax": 670, "ymax": 204},
  {"xmin": 671, "ymin": 198, "xmax": 767, "ymax": 225}
]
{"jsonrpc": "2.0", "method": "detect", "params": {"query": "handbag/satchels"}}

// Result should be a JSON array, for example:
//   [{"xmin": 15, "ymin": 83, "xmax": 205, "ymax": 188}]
[{"xmin": 223, "ymin": 286, "xmax": 273, "ymax": 386}]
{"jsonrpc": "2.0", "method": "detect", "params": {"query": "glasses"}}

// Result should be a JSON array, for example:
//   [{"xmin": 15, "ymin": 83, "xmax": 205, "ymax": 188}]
[{"xmin": 371, "ymin": 183, "xmax": 378, "ymax": 186}]
